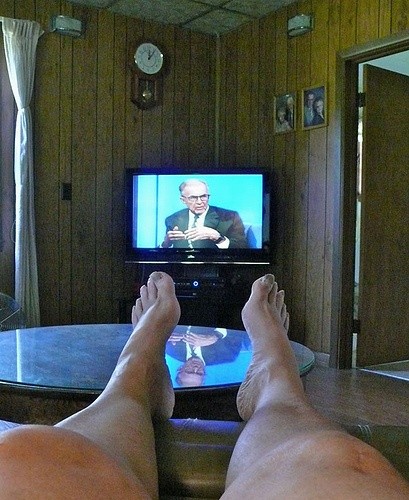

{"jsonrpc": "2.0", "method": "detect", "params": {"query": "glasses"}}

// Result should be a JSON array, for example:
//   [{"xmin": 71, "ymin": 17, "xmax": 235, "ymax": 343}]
[
  {"xmin": 181, "ymin": 194, "xmax": 210, "ymax": 202},
  {"xmin": 177, "ymin": 368, "xmax": 205, "ymax": 376}
]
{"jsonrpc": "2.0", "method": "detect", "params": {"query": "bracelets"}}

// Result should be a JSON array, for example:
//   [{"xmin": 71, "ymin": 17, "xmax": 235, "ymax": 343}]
[
  {"xmin": 215, "ymin": 234, "xmax": 226, "ymax": 244},
  {"xmin": 214, "ymin": 331, "xmax": 223, "ymax": 340}
]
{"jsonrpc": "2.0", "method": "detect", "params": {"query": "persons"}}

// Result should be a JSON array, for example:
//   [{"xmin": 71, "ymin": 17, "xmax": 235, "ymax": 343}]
[
  {"xmin": 305, "ymin": 91, "xmax": 325, "ymax": 125},
  {"xmin": 156, "ymin": 179, "xmax": 249, "ymax": 249},
  {"xmin": 1, "ymin": 272, "xmax": 409, "ymax": 499},
  {"xmin": 164, "ymin": 324, "xmax": 243, "ymax": 387},
  {"xmin": 278, "ymin": 97, "xmax": 294, "ymax": 132}
]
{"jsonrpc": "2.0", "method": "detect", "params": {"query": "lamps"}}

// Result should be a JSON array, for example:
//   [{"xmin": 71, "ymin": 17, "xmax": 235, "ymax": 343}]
[
  {"xmin": 286, "ymin": 12, "xmax": 315, "ymax": 39},
  {"xmin": 273, "ymin": 89, "xmax": 298, "ymax": 135},
  {"xmin": 301, "ymin": 82, "xmax": 329, "ymax": 131},
  {"xmin": 50, "ymin": 14, "xmax": 87, "ymax": 40}
]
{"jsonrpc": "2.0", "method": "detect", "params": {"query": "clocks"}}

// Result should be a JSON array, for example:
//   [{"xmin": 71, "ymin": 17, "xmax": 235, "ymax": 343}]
[{"xmin": 129, "ymin": 37, "xmax": 166, "ymax": 111}]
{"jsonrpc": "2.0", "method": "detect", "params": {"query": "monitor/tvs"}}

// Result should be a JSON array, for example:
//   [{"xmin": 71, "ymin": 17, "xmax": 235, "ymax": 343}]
[{"xmin": 125, "ymin": 167, "xmax": 271, "ymax": 267}]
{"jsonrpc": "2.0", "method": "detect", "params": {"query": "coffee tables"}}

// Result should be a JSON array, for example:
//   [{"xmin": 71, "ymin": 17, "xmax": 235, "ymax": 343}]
[{"xmin": 0, "ymin": 323, "xmax": 317, "ymax": 400}]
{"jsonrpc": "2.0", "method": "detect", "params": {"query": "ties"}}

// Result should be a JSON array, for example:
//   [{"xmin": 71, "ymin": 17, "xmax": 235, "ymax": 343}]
[
  {"xmin": 191, "ymin": 215, "xmax": 199, "ymax": 229},
  {"xmin": 189, "ymin": 343, "xmax": 197, "ymax": 358}
]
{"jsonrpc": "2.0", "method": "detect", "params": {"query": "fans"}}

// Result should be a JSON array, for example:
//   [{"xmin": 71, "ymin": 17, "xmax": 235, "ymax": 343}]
[{"xmin": 0, "ymin": 294, "xmax": 26, "ymax": 330}]
